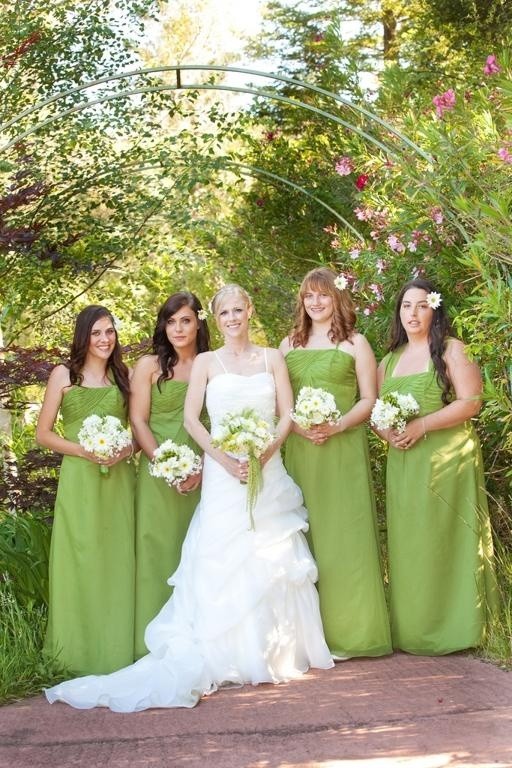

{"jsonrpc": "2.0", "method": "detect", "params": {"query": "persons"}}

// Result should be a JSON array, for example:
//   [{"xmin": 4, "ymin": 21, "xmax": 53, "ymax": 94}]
[
  {"xmin": 38, "ymin": 306, "xmax": 142, "ymax": 673},
  {"xmin": 273, "ymin": 270, "xmax": 393, "ymax": 659},
  {"xmin": 182, "ymin": 282, "xmax": 296, "ymax": 684},
  {"xmin": 368, "ymin": 277, "xmax": 502, "ymax": 656},
  {"xmin": 130, "ymin": 290, "xmax": 211, "ymax": 665}
]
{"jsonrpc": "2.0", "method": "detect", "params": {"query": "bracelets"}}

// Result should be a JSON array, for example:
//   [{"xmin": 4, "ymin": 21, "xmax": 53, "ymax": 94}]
[
  {"xmin": 126, "ymin": 441, "xmax": 133, "ymax": 464},
  {"xmin": 422, "ymin": 414, "xmax": 429, "ymax": 441}
]
